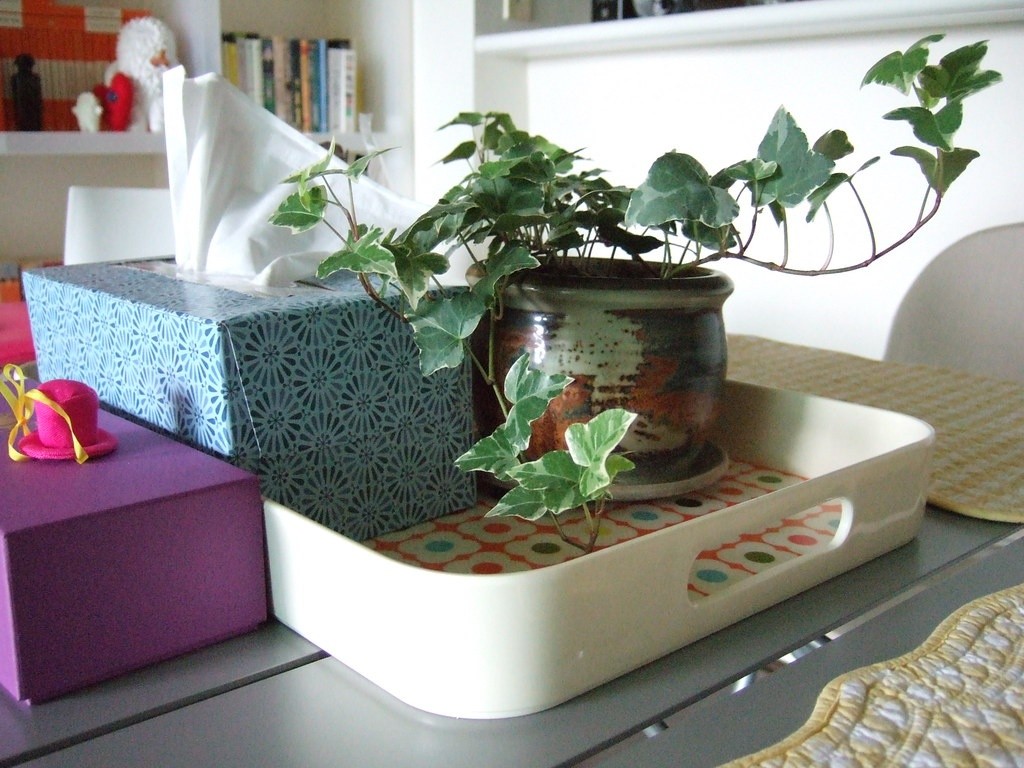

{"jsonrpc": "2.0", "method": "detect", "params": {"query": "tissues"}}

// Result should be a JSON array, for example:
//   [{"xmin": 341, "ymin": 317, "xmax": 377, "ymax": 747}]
[{"xmin": 22, "ymin": 66, "xmax": 479, "ymax": 543}]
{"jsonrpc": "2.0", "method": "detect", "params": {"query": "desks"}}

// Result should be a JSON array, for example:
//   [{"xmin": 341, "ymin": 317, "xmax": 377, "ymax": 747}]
[{"xmin": 1, "ymin": 507, "xmax": 1024, "ymax": 768}]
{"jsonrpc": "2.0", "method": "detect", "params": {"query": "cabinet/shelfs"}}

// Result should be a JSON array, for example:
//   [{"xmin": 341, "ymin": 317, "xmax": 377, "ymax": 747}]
[{"xmin": 0, "ymin": 0, "xmax": 414, "ymax": 298}]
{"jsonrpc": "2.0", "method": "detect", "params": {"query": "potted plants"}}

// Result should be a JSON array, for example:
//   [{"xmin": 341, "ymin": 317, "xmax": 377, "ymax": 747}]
[{"xmin": 270, "ymin": 34, "xmax": 1002, "ymax": 551}]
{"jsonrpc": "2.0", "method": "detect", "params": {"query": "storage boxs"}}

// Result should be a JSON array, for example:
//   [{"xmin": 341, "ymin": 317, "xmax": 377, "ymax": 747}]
[{"xmin": 0, "ymin": 376, "xmax": 268, "ymax": 707}]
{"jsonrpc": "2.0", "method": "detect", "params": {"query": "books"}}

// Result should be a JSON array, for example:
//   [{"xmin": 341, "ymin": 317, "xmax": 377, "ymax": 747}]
[{"xmin": 222, "ymin": 32, "xmax": 359, "ymax": 135}]
